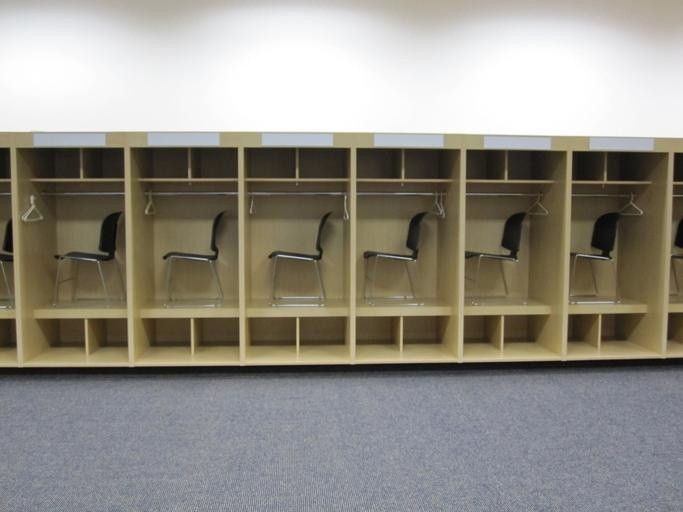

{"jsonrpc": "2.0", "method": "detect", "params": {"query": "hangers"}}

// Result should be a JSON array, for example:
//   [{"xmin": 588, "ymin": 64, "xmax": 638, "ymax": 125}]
[
  {"xmin": 21, "ymin": 195, "xmax": 44, "ymax": 224},
  {"xmin": 428, "ymin": 191, "xmax": 446, "ymax": 219},
  {"xmin": 526, "ymin": 191, "xmax": 549, "ymax": 216},
  {"xmin": 342, "ymin": 196, "xmax": 349, "ymax": 221},
  {"xmin": 144, "ymin": 189, "xmax": 158, "ymax": 216},
  {"xmin": 249, "ymin": 191, "xmax": 257, "ymax": 214},
  {"xmin": 618, "ymin": 192, "xmax": 644, "ymax": 216}
]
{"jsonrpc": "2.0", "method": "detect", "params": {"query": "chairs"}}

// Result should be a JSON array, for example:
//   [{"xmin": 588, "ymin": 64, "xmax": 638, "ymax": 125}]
[
  {"xmin": 52, "ymin": 211, "xmax": 128, "ymax": 309},
  {"xmin": 267, "ymin": 211, "xmax": 333, "ymax": 308},
  {"xmin": 362, "ymin": 211, "xmax": 430, "ymax": 307},
  {"xmin": 0, "ymin": 217, "xmax": 15, "ymax": 309},
  {"xmin": 465, "ymin": 212, "xmax": 527, "ymax": 305},
  {"xmin": 671, "ymin": 217, "xmax": 683, "ymax": 303},
  {"xmin": 569, "ymin": 212, "xmax": 624, "ymax": 305},
  {"xmin": 162, "ymin": 211, "xmax": 228, "ymax": 309}
]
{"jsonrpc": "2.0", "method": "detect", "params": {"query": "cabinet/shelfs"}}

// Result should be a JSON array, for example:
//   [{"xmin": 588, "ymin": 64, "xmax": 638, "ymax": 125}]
[{"xmin": 0, "ymin": 129, "xmax": 683, "ymax": 372}]
